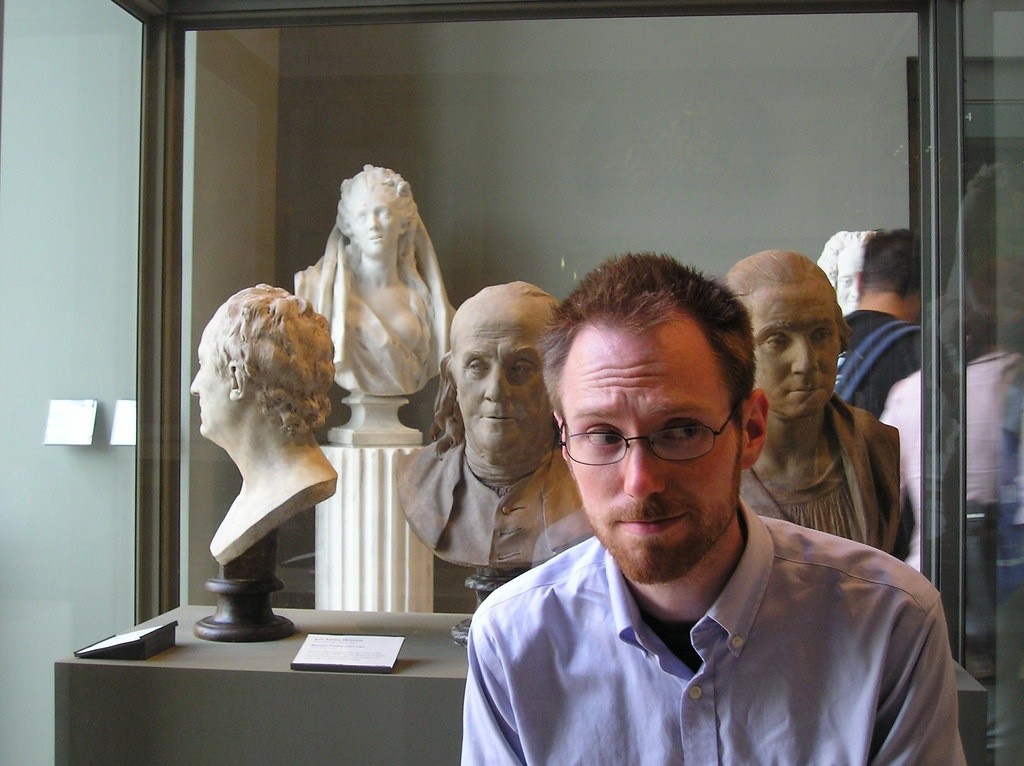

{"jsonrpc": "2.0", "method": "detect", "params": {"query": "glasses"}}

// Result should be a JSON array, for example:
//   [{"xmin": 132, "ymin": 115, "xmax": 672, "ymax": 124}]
[{"xmin": 557, "ymin": 401, "xmax": 742, "ymax": 467}]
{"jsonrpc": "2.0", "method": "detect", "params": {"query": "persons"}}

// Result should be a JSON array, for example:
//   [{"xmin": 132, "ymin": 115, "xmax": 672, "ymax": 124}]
[
  {"xmin": 294, "ymin": 164, "xmax": 456, "ymax": 396},
  {"xmin": 833, "ymin": 229, "xmax": 1024, "ymax": 766},
  {"xmin": 395, "ymin": 279, "xmax": 596, "ymax": 569},
  {"xmin": 461, "ymin": 254, "xmax": 967, "ymax": 766},
  {"xmin": 720, "ymin": 231, "xmax": 900, "ymax": 554},
  {"xmin": 190, "ymin": 283, "xmax": 338, "ymax": 565}
]
